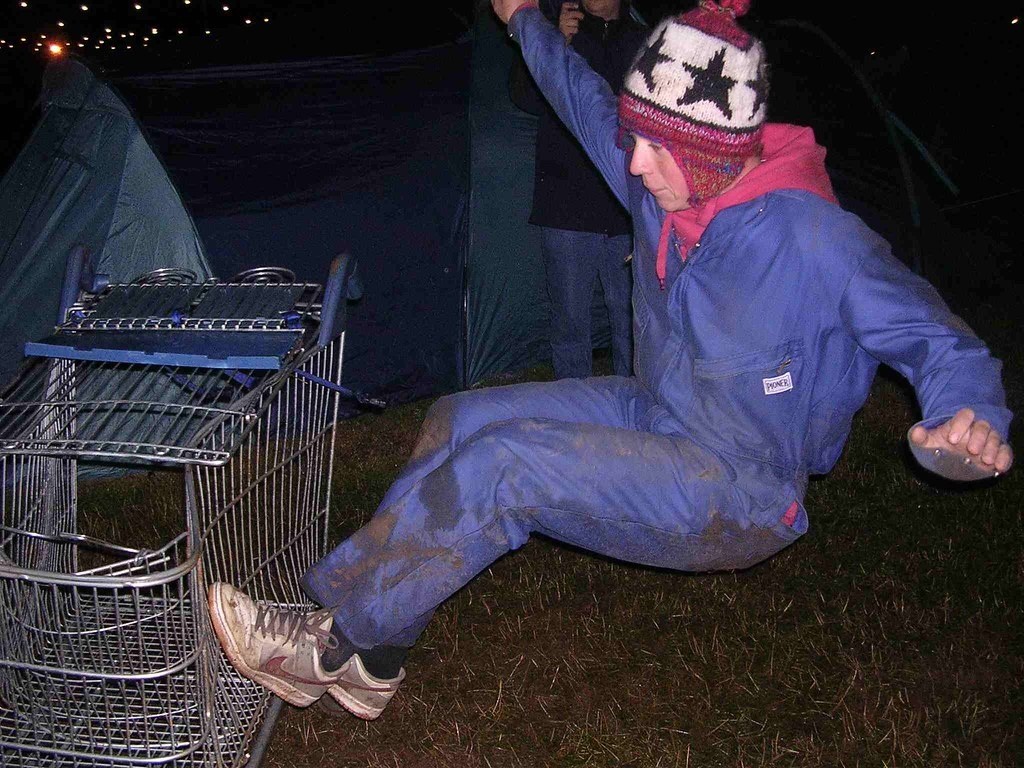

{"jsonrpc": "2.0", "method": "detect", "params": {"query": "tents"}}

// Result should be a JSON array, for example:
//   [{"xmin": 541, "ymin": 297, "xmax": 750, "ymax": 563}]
[{"xmin": 0, "ymin": 41, "xmax": 638, "ymax": 482}]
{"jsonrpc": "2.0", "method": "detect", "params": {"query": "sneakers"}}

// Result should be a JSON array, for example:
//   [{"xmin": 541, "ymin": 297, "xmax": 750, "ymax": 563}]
[
  {"xmin": 327, "ymin": 653, "xmax": 406, "ymax": 720},
  {"xmin": 209, "ymin": 581, "xmax": 351, "ymax": 708}
]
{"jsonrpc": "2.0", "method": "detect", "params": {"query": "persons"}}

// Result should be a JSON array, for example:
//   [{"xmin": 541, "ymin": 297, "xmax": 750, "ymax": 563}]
[
  {"xmin": 207, "ymin": 0, "xmax": 1013, "ymax": 721},
  {"xmin": 506, "ymin": 0, "xmax": 652, "ymax": 379}
]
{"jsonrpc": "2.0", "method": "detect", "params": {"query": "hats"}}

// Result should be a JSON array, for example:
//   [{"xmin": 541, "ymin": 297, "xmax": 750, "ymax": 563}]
[{"xmin": 616, "ymin": 0, "xmax": 769, "ymax": 206}]
